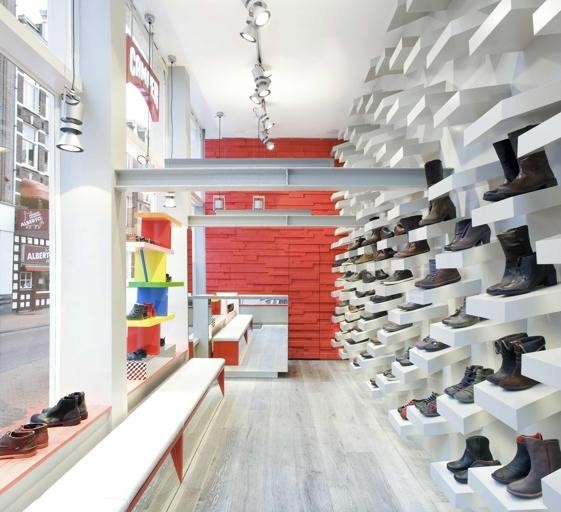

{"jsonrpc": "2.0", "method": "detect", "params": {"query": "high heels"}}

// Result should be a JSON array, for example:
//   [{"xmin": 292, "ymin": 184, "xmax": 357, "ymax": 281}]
[
  {"xmin": 451, "ymin": 222, "xmax": 491, "ymax": 251},
  {"xmin": 444, "ymin": 218, "xmax": 472, "ymax": 251}
]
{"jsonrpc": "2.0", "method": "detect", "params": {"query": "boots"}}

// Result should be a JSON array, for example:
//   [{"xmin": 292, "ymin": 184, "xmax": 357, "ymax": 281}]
[
  {"xmin": 454, "ymin": 368, "xmax": 494, "ymax": 403},
  {"xmin": 421, "ymin": 158, "xmax": 456, "ymax": 224},
  {"xmin": 143, "ymin": 303, "xmax": 152, "ymax": 319},
  {"xmin": 497, "ymin": 124, "xmax": 557, "ymax": 195},
  {"xmin": 127, "ymin": 304, "xmax": 144, "ymax": 320},
  {"xmin": 444, "ymin": 365, "xmax": 483, "ymax": 398},
  {"xmin": 507, "ymin": 439, "xmax": 561, "ymax": 499},
  {"xmin": 491, "ymin": 432, "xmax": 542, "ymax": 484},
  {"xmin": 483, "ymin": 138, "xmax": 519, "ymax": 201},
  {"xmin": 486, "ymin": 333, "xmax": 528, "ymax": 384},
  {"xmin": 42, "ymin": 392, "xmax": 88, "ymax": 420},
  {"xmin": 447, "ymin": 436, "xmax": 493, "ymax": 472},
  {"xmin": 362, "ymin": 216, "xmax": 380, "ymax": 245},
  {"xmin": 419, "ymin": 159, "xmax": 443, "ymax": 226},
  {"xmin": 500, "ymin": 252, "xmax": 557, "ymax": 295},
  {"xmin": 486, "ymin": 225, "xmax": 533, "ymax": 295},
  {"xmin": 500, "ymin": 336, "xmax": 546, "ymax": 390},
  {"xmin": 394, "ymin": 215, "xmax": 430, "ymax": 258},
  {"xmin": 143, "ymin": 306, "xmax": 147, "ymax": 319},
  {"xmin": 454, "ymin": 461, "xmax": 501, "ymax": 484},
  {"xmin": 31, "ymin": 397, "xmax": 81, "ymax": 427}
]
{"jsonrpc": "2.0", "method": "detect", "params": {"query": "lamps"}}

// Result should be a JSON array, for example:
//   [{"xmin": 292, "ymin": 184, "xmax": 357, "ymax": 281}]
[
  {"xmin": 136, "ymin": 12, "xmax": 156, "ymax": 169},
  {"xmin": 212, "ymin": 111, "xmax": 226, "ymax": 214},
  {"xmin": 163, "ymin": 54, "xmax": 176, "ymax": 208},
  {"xmin": 252, "ymin": 122, "xmax": 265, "ymax": 212},
  {"xmin": 240, "ymin": 0, "xmax": 275, "ymax": 151},
  {"xmin": 57, "ymin": 1, "xmax": 85, "ymax": 154}
]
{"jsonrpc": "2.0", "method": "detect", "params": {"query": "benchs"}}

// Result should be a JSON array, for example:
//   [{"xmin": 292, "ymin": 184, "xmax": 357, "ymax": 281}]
[
  {"xmin": 211, "ymin": 314, "xmax": 255, "ymax": 367},
  {"xmin": 22, "ymin": 356, "xmax": 226, "ymax": 512}
]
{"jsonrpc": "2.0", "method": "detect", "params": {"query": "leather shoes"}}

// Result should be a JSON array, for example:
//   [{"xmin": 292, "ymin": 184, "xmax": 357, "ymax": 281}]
[
  {"xmin": 419, "ymin": 268, "xmax": 461, "ymax": 289},
  {"xmin": 383, "ymin": 322, "xmax": 413, "ymax": 332},
  {"xmin": 348, "ymin": 238, "xmax": 364, "ymax": 250},
  {"xmin": 370, "ymin": 294, "xmax": 402, "ymax": 303},
  {"xmin": 446, "ymin": 298, "xmax": 483, "ymax": 328},
  {"xmin": 355, "ymin": 290, "xmax": 375, "ymax": 297},
  {"xmin": 0, "ymin": 430, "xmax": 37, "ymax": 458},
  {"xmin": 415, "ymin": 276, "xmax": 428, "ymax": 287},
  {"xmin": 375, "ymin": 269, "xmax": 388, "ymax": 280},
  {"xmin": 380, "ymin": 227, "xmax": 393, "ymax": 239},
  {"xmin": 396, "ymin": 347, "xmax": 411, "ymax": 361},
  {"xmin": 400, "ymin": 359, "xmax": 413, "ymax": 366},
  {"xmin": 383, "ymin": 270, "xmax": 413, "ymax": 285},
  {"xmin": 362, "ymin": 271, "xmax": 375, "ymax": 283},
  {"xmin": 396, "ymin": 301, "xmax": 432, "ymax": 310},
  {"xmin": 424, "ymin": 341, "xmax": 449, "ymax": 351},
  {"xmin": 394, "ymin": 225, "xmax": 405, "ymax": 235},
  {"xmin": 15, "ymin": 423, "xmax": 48, "ymax": 448},
  {"xmin": 360, "ymin": 312, "xmax": 387, "ymax": 320},
  {"xmin": 416, "ymin": 337, "xmax": 434, "ymax": 349},
  {"xmin": 442, "ymin": 304, "xmax": 463, "ymax": 325}
]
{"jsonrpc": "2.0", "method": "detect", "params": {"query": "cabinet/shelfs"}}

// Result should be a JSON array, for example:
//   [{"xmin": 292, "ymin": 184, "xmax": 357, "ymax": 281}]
[
  {"xmin": 331, "ymin": 0, "xmax": 561, "ymax": 512},
  {"xmin": 126, "ymin": 210, "xmax": 188, "ymax": 395}
]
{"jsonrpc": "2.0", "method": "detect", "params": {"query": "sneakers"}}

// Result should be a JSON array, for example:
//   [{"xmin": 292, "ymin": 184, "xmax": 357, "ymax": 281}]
[
  {"xmin": 420, "ymin": 400, "xmax": 439, "ymax": 417},
  {"xmin": 374, "ymin": 251, "xmax": 386, "ymax": 260},
  {"xmin": 331, "ymin": 299, "xmax": 364, "ymax": 360},
  {"xmin": 414, "ymin": 393, "xmax": 438, "ymax": 409},
  {"xmin": 355, "ymin": 254, "xmax": 374, "ymax": 264},
  {"xmin": 397, "ymin": 400, "xmax": 415, "ymax": 420},
  {"xmin": 331, "ymin": 257, "xmax": 357, "ymax": 291},
  {"xmin": 382, "ymin": 369, "xmax": 398, "ymax": 380},
  {"xmin": 350, "ymin": 331, "xmax": 380, "ymax": 391},
  {"xmin": 385, "ymin": 248, "xmax": 396, "ymax": 257}
]
